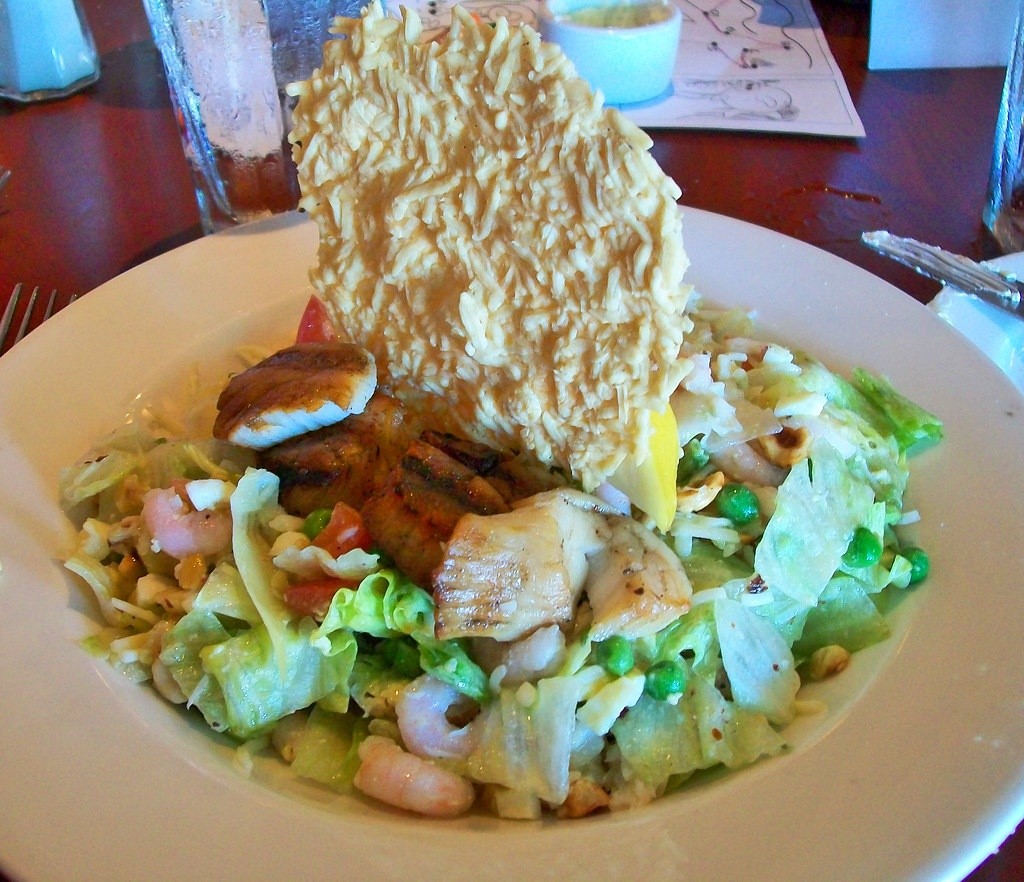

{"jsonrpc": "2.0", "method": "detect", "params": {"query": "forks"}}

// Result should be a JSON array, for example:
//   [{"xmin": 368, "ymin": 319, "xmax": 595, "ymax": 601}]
[{"xmin": 0, "ymin": 282, "xmax": 79, "ymax": 356}]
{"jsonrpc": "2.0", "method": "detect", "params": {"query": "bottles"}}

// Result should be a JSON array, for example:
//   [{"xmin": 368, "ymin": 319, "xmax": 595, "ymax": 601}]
[{"xmin": 1, "ymin": 0, "xmax": 99, "ymax": 102}]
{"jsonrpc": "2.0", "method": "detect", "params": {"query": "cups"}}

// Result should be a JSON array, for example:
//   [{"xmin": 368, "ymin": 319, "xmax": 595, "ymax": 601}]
[{"xmin": 141, "ymin": 0, "xmax": 388, "ymax": 234}]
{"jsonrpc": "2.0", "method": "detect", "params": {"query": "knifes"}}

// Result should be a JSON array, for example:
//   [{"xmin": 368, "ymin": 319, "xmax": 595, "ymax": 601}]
[{"xmin": 858, "ymin": 228, "xmax": 1022, "ymax": 314}]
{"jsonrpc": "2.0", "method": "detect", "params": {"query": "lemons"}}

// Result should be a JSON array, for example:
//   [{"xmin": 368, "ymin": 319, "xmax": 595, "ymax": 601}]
[{"xmin": 610, "ymin": 403, "xmax": 681, "ymax": 533}]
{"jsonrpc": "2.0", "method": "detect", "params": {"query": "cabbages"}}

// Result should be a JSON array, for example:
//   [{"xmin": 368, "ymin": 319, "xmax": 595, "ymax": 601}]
[{"xmin": 53, "ymin": 302, "xmax": 946, "ymax": 821}]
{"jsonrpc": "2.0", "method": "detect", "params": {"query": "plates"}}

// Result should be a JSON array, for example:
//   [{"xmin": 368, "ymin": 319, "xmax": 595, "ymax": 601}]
[
  {"xmin": 0, "ymin": 212, "xmax": 1023, "ymax": 882},
  {"xmin": 926, "ymin": 251, "xmax": 1023, "ymax": 391}
]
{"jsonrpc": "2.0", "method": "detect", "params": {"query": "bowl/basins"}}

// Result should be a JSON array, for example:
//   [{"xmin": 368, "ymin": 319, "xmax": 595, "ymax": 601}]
[{"xmin": 540, "ymin": 0, "xmax": 681, "ymax": 105}]
